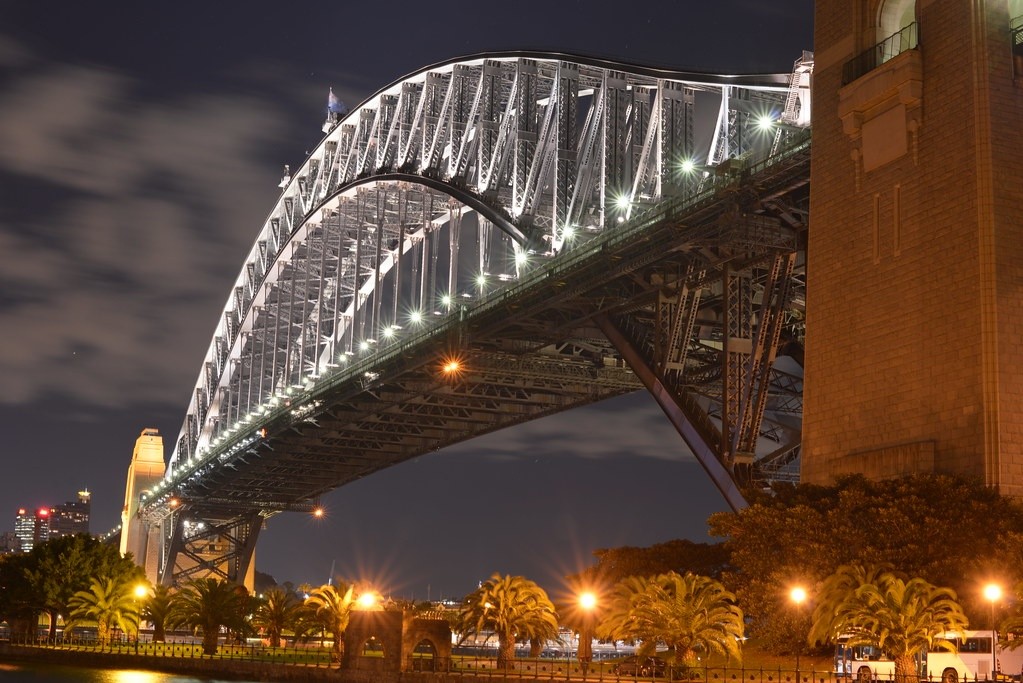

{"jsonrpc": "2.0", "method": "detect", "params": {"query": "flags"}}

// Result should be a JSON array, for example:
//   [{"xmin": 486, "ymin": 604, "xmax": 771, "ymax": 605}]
[{"xmin": 329, "ymin": 93, "xmax": 348, "ymax": 114}]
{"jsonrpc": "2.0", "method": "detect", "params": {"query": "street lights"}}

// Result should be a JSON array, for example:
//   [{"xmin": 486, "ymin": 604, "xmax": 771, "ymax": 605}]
[
  {"xmin": 984, "ymin": 585, "xmax": 999, "ymax": 630},
  {"xmin": 580, "ymin": 591, "xmax": 596, "ymax": 683},
  {"xmin": 134, "ymin": 584, "xmax": 146, "ymax": 654},
  {"xmin": 790, "ymin": 587, "xmax": 805, "ymax": 683}
]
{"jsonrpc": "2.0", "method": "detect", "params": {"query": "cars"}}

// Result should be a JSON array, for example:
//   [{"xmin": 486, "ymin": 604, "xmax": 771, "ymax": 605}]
[{"xmin": 612, "ymin": 655, "xmax": 668, "ymax": 677}]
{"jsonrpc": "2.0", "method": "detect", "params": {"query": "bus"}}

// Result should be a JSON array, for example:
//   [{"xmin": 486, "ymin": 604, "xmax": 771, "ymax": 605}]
[{"xmin": 833, "ymin": 630, "xmax": 999, "ymax": 683}]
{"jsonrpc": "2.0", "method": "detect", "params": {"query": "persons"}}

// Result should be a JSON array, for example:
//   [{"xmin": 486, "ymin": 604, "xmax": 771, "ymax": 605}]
[
  {"xmin": 642, "ymin": 657, "xmax": 652, "ymax": 676},
  {"xmin": 879, "ymin": 650, "xmax": 889, "ymax": 660}
]
